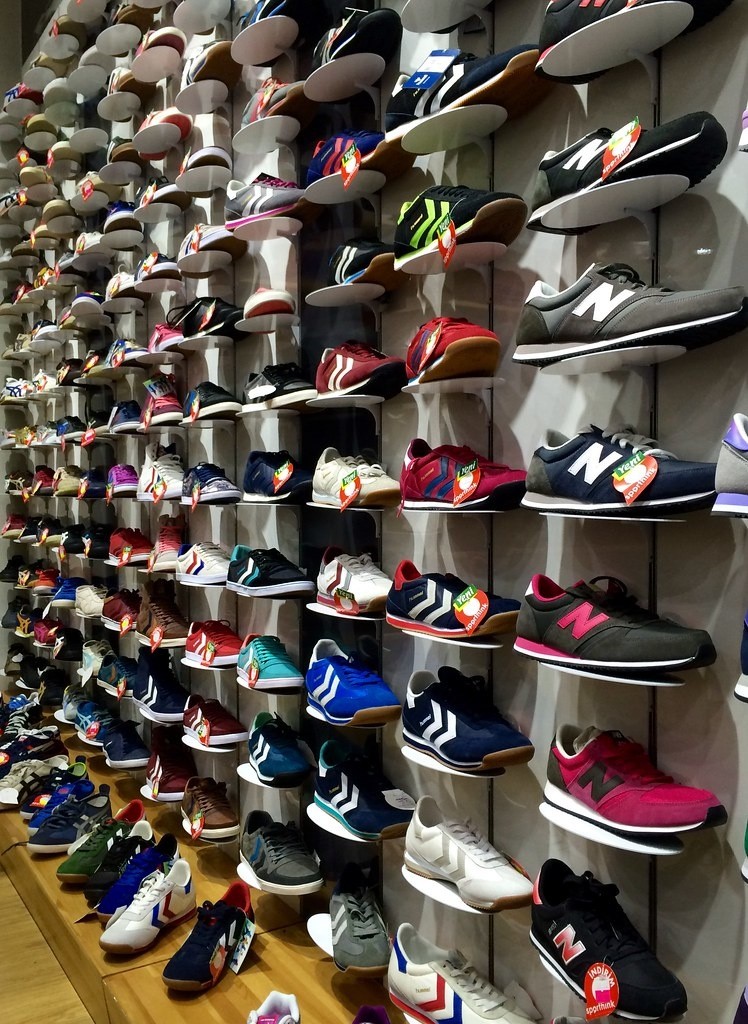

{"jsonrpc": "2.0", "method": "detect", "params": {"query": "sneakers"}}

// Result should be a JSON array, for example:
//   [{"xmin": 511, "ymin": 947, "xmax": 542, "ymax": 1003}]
[
  {"xmin": 384, "ymin": 559, "xmax": 521, "ymax": 639},
  {"xmin": 526, "ymin": 110, "xmax": 728, "ymax": 237},
  {"xmin": 734, "ymin": 606, "xmax": 748, "ymax": 704},
  {"xmin": 710, "ymin": 412, "xmax": 748, "ymax": 518},
  {"xmin": 510, "ymin": 260, "xmax": 748, "ymax": 367},
  {"xmin": 392, "ymin": 185, "xmax": 529, "ymax": 272},
  {"xmin": 405, "ymin": 315, "xmax": 504, "ymax": 385},
  {"xmin": 401, "ymin": 669, "xmax": 535, "ymax": 772},
  {"xmin": 2, "ymin": 1, "xmax": 404, "ymax": 1024},
  {"xmin": 384, "ymin": 43, "xmax": 553, "ymax": 158},
  {"xmin": 512, "ymin": 573, "xmax": 718, "ymax": 674},
  {"xmin": 395, "ymin": 437, "xmax": 528, "ymax": 520},
  {"xmin": 529, "ymin": 859, "xmax": 689, "ymax": 1023},
  {"xmin": 387, "ymin": 923, "xmax": 538, "ymax": 1024},
  {"xmin": 518, "ymin": 422, "xmax": 718, "ymax": 517},
  {"xmin": 550, "ymin": 1015, "xmax": 587, "ymax": 1024},
  {"xmin": 534, "ymin": 0, "xmax": 735, "ymax": 85},
  {"xmin": 402, "ymin": 794, "xmax": 535, "ymax": 913},
  {"xmin": 542, "ymin": 722, "xmax": 728, "ymax": 838}
]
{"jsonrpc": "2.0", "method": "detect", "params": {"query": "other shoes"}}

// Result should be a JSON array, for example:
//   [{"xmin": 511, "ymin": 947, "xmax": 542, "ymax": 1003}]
[{"xmin": 399, "ymin": 0, "xmax": 496, "ymax": 35}]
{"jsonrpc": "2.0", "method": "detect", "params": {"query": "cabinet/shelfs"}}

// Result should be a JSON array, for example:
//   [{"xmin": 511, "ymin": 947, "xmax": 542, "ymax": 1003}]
[{"xmin": 0, "ymin": 0, "xmax": 748, "ymax": 1024}]
